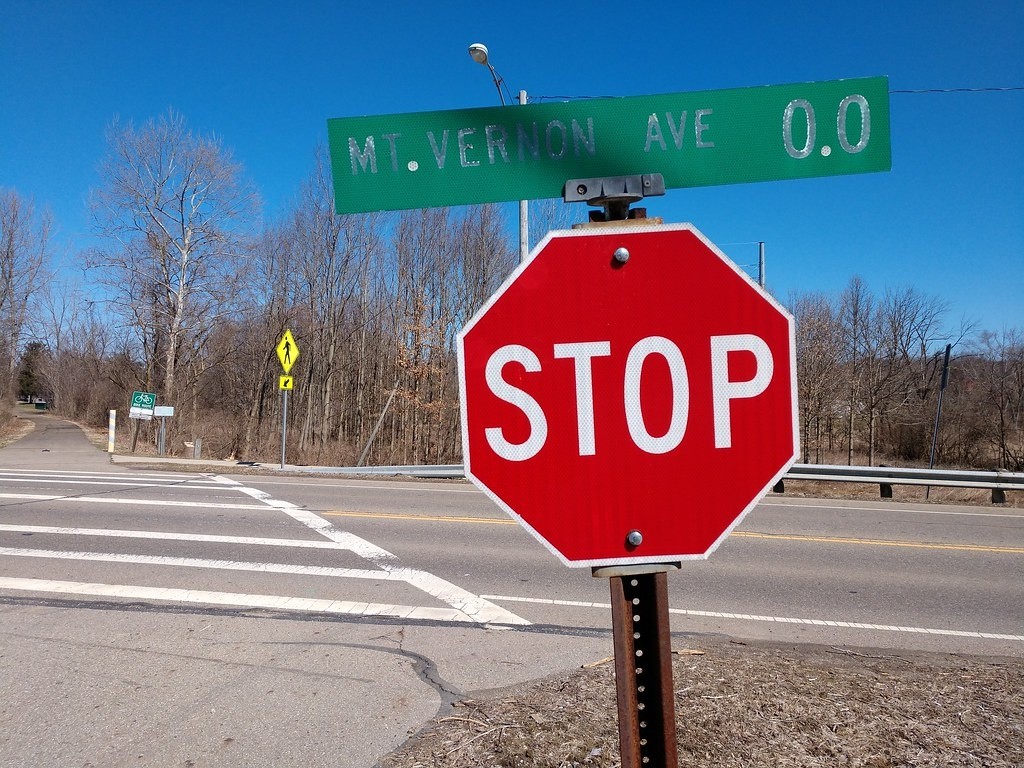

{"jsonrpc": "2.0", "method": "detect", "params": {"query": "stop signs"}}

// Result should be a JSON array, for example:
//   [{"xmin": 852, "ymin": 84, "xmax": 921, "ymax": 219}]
[{"xmin": 457, "ymin": 227, "xmax": 803, "ymax": 566}]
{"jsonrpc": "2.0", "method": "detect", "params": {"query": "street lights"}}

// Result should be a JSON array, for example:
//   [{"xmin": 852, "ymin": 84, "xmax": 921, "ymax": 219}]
[{"xmin": 468, "ymin": 43, "xmax": 528, "ymax": 264}]
